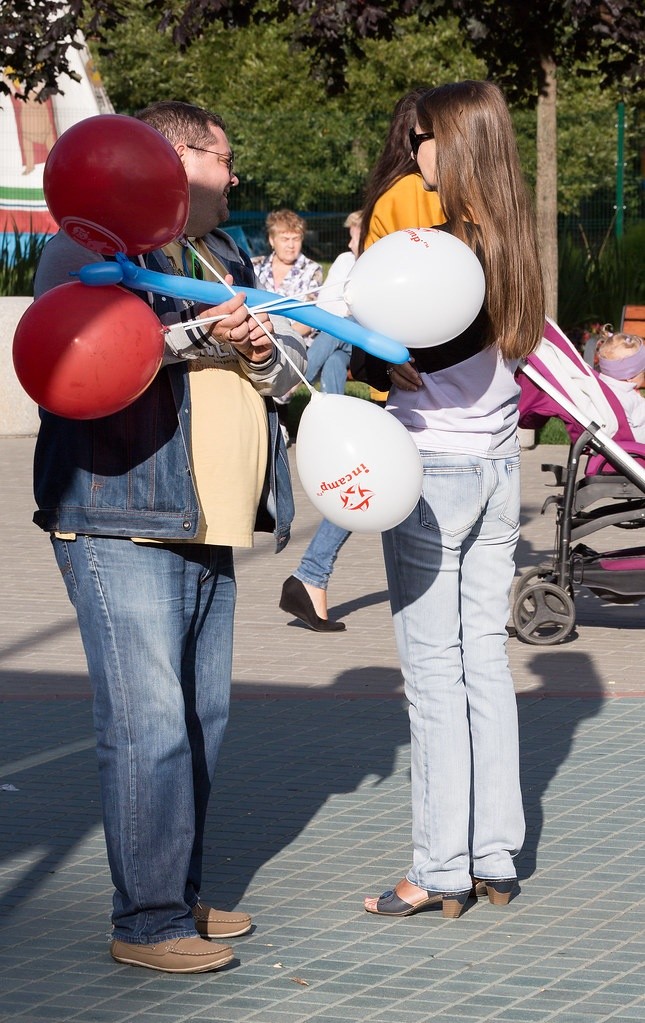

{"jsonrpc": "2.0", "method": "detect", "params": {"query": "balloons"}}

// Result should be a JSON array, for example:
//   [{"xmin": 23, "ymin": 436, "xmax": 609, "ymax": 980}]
[
  {"xmin": 294, "ymin": 390, "xmax": 422, "ymax": 534},
  {"xmin": 44, "ymin": 114, "xmax": 190, "ymax": 255},
  {"xmin": 12, "ymin": 278, "xmax": 165, "ymax": 421},
  {"xmin": 343, "ymin": 227, "xmax": 486, "ymax": 349},
  {"xmin": 66, "ymin": 253, "xmax": 409, "ymax": 365}
]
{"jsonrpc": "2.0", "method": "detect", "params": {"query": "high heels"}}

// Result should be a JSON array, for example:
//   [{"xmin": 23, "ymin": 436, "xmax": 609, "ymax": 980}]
[
  {"xmin": 365, "ymin": 888, "xmax": 471, "ymax": 919},
  {"xmin": 470, "ymin": 878, "xmax": 516, "ymax": 906},
  {"xmin": 279, "ymin": 575, "xmax": 346, "ymax": 632}
]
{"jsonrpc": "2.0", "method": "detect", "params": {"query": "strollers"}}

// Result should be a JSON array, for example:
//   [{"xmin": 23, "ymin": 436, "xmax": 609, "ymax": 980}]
[{"xmin": 509, "ymin": 313, "xmax": 645, "ymax": 645}]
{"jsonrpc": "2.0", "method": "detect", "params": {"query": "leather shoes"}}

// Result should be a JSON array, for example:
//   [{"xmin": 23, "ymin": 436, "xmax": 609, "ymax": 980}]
[
  {"xmin": 192, "ymin": 902, "xmax": 252, "ymax": 938},
  {"xmin": 110, "ymin": 938, "xmax": 234, "ymax": 974}
]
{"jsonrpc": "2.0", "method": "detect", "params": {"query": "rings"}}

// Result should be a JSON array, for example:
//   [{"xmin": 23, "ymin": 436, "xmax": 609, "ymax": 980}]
[
  {"xmin": 228, "ymin": 329, "xmax": 234, "ymax": 339},
  {"xmin": 385, "ymin": 368, "xmax": 394, "ymax": 376}
]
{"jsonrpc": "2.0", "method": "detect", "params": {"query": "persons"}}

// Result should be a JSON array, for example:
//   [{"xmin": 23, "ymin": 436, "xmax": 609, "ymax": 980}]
[
  {"xmin": 272, "ymin": 211, "xmax": 363, "ymax": 405},
  {"xmin": 278, "ymin": 92, "xmax": 448, "ymax": 634},
  {"xmin": 351, "ymin": 79, "xmax": 528, "ymax": 918},
  {"xmin": 252, "ymin": 208, "xmax": 322, "ymax": 445},
  {"xmin": 32, "ymin": 102, "xmax": 307, "ymax": 973},
  {"xmin": 595, "ymin": 324, "xmax": 645, "ymax": 435}
]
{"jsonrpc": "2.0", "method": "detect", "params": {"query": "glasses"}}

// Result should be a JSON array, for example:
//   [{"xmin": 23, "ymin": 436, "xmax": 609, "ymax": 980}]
[
  {"xmin": 187, "ymin": 145, "xmax": 234, "ymax": 175},
  {"xmin": 181, "ymin": 243, "xmax": 204, "ymax": 281},
  {"xmin": 409, "ymin": 128, "xmax": 434, "ymax": 154}
]
